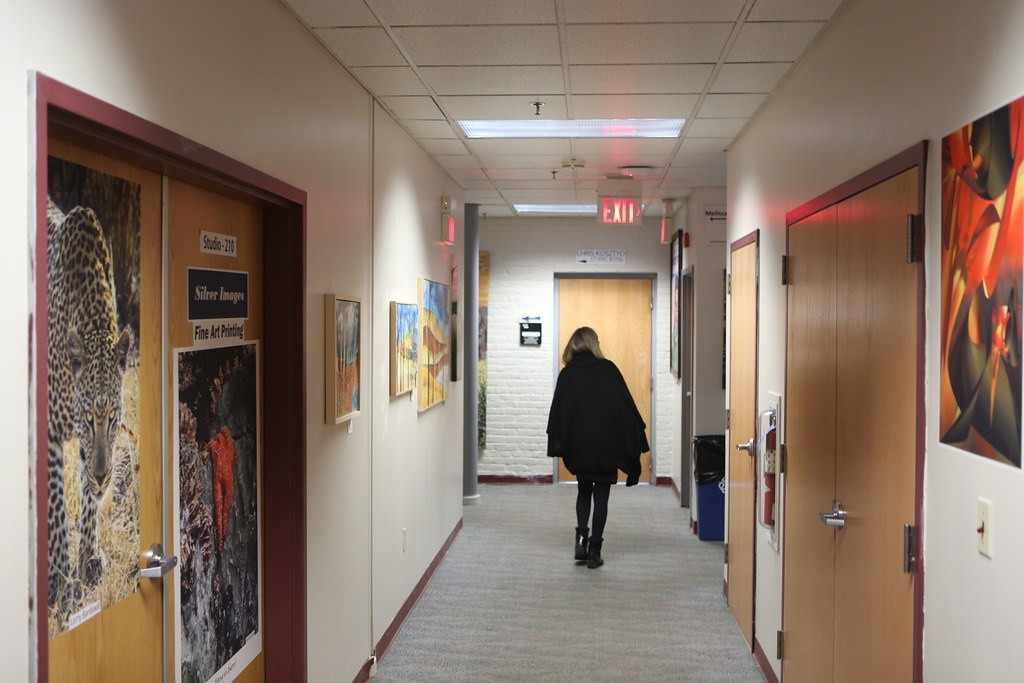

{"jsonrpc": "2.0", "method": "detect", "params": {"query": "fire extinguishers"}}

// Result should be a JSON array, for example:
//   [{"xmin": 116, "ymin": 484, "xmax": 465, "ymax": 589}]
[{"xmin": 762, "ymin": 411, "xmax": 777, "ymax": 526}]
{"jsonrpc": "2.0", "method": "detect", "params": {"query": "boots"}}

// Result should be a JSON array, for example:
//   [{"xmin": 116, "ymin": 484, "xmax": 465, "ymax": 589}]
[
  {"xmin": 574, "ymin": 526, "xmax": 589, "ymax": 560},
  {"xmin": 587, "ymin": 536, "xmax": 604, "ymax": 569}
]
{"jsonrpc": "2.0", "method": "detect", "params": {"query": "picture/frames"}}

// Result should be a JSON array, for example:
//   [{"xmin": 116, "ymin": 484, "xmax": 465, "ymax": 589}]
[
  {"xmin": 390, "ymin": 300, "xmax": 418, "ymax": 396},
  {"xmin": 325, "ymin": 293, "xmax": 362, "ymax": 424},
  {"xmin": 670, "ymin": 229, "xmax": 683, "ymax": 379},
  {"xmin": 417, "ymin": 276, "xmax": 451, "ymax": 412}
]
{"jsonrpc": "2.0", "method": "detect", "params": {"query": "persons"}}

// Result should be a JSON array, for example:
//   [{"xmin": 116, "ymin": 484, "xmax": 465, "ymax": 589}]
[{"xmin": 546, "ymin": 327, "xmax": 650, "ymax": 568}]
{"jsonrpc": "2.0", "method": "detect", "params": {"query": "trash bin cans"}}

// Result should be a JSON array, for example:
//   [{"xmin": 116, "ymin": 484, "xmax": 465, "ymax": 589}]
[{"xmin": 693, "ymin": 434, "xmax": 725, "ymax": 541}]
